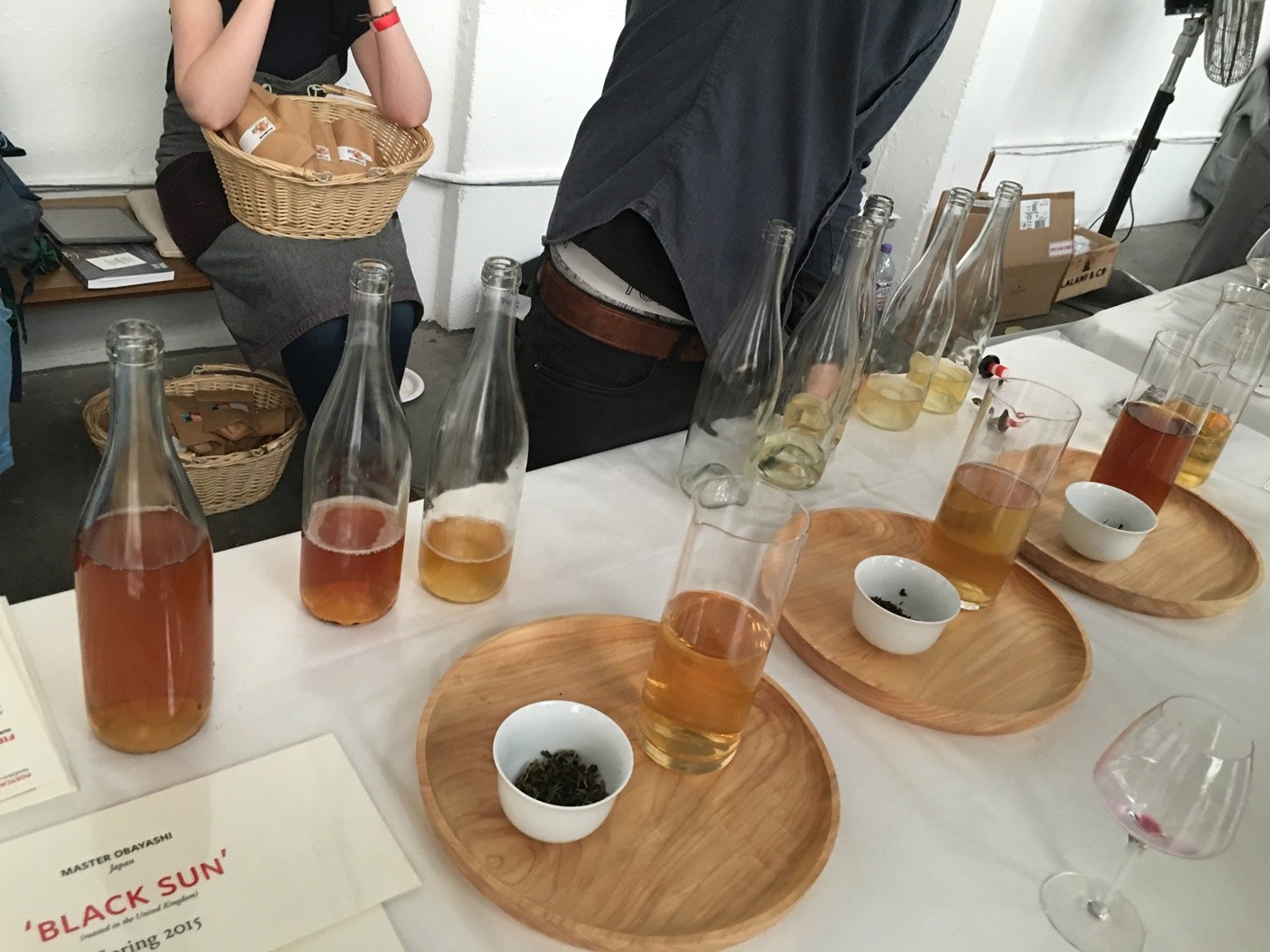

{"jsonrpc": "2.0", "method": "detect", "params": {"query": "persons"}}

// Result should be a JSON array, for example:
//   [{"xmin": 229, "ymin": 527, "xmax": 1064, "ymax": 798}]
[
  {"xmin": 155, "ymin": 0, "xmax": 432, "ymax": 531},
  {"xmin": 477, "ymin": 0, "xmax": 960, "ymax": 484}
]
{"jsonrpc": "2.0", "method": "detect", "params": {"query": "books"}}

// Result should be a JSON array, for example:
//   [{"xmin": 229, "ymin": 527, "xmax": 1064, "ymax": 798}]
[{"xmin": 43, "ymin": 233, "xmax": 175, "ymax": 289}]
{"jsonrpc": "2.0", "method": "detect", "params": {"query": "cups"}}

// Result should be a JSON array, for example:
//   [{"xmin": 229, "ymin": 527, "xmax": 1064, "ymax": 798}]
[
  {"xmin": 924, "ymin": 376, "xmax": 1083, "ymax": 610},
  {"xmin": 1087, "ymin": 327, "xmax": 1235, "ymax": 517},
  {"xmin": 1063, "ymin": 481, "xmax": 1159, "ymax": 562},
  {"xmin": 636, "ymin": 476, "xmax": 810, "ymax": 774},
  {"xmin": 492, "ymin": 702, "xmax": 634, "ymax": 845},
  {"xmin": 851, "ymin": 553, "xmax": 965, "ymax": 655}
]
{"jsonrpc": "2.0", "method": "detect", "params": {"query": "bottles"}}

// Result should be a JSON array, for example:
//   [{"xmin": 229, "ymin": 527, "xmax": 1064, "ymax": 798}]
[
  {"xmin": 1163, "ymin": 256, "xmax": 1270, "ymax": 493},
  {"xmin": 850, "ymin": 188, "xmax": 974, "ymax": 432},
  {"xmin": 877, "ymin": 243, "xmax": 896, "ymax": 326},
  {"xmin": 679, "ymin": 220, "xmax": 796, "ymax": 509},
  {"xmin": 909, "ymin": 182, "xmax": 1021, "ymax": 413},
  {"xmin": 301, "ymin": 259, "xmax": 412, "ymax": 624},
  {"xmin": 73, "ymin": 319, "xmax": 214, "ymax": 754},
  {"xmin": 757, "ymin": 217, "xmax": 876, "ymax": 489},
  {"xmin": 418, "ymin": 259, "xmax": 529, "ymax": 603},
  {"xmin": 831, "ymin": 193, "xmax": 893, "ymax": 451}
]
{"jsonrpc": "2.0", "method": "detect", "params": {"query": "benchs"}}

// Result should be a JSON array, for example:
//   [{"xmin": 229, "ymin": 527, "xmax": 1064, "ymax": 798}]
[{"xmin": 10, "ymin": 196, "xmax": 223, "ymax": 305}]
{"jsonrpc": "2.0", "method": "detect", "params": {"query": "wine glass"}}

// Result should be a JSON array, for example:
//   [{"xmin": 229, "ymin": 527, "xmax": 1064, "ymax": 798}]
[
  {"xmin": 1039, "ymin": 696, "xmax": 1255, "ymax": 952},
  {"xmin": 1244, "ymin": 228, "xmax": 1270, "ymax": 290}
]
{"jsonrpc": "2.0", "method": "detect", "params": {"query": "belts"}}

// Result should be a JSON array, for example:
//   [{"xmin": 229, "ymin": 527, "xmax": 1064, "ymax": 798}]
[{"xmin": 535, "ymin": 253, "xmax": 710, "ymax": 364}]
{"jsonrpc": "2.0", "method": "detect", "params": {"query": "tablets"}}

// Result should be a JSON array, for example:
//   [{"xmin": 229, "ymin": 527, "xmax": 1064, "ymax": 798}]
[{"xmin": 39, "ymin": 205, "xmax": 157, "ymax": 246}]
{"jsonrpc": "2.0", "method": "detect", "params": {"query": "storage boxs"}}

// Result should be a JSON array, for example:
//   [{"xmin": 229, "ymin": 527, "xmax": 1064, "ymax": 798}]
[
  {"xmin": 1055, "ymin": 227, "xmax": 1119, "ymax": 303},
  {"xmin": 923, "ymin": 191, "xmax": 1074, "ymax": 323}
]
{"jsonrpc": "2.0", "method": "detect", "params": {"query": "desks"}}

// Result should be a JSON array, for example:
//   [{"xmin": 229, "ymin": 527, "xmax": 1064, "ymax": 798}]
[{"xmin": 3, "ymin": 264, "xmax": 1269, "ymax": 950}]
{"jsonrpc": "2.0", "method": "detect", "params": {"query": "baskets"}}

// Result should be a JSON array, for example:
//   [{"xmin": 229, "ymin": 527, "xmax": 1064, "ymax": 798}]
[
  {"xmin": 198, "ymin": 83, "xmax": 434, "ymax": 240},
  {"xmin": 82, "ymin": 362, "xmax": 305, "ymax": 516}
]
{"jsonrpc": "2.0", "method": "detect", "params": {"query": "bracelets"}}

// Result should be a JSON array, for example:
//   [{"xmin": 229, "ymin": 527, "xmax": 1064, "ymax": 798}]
[{"xmin": 354, "ymin": 6, "xmax": 400, "ymax": 32}]
